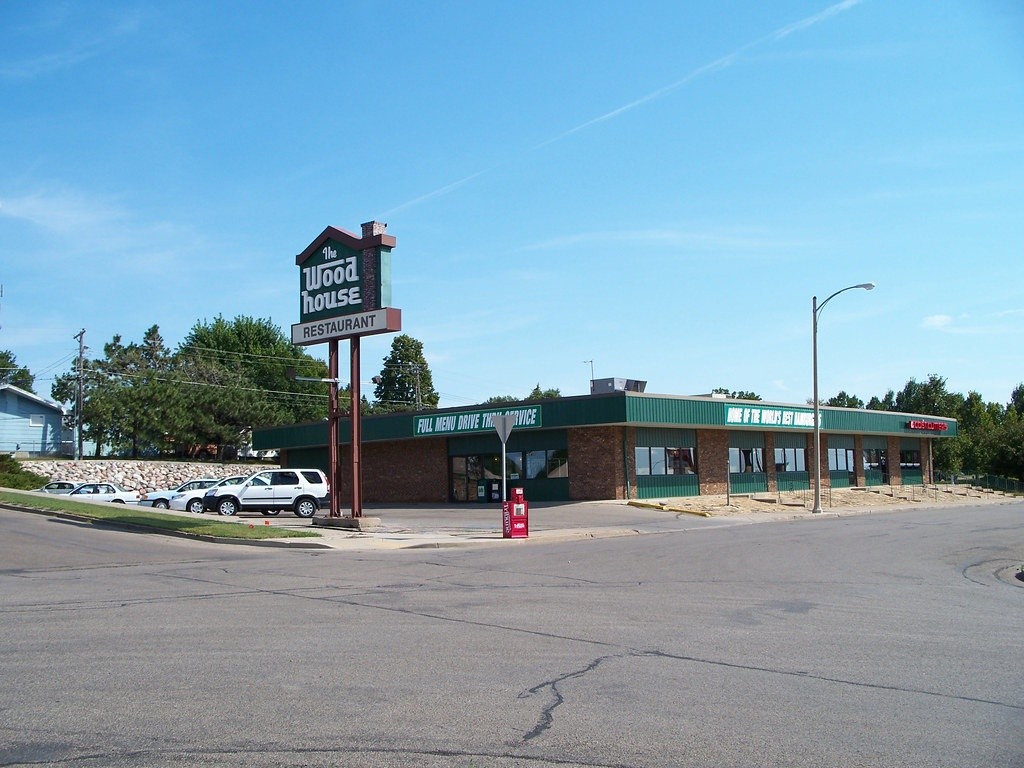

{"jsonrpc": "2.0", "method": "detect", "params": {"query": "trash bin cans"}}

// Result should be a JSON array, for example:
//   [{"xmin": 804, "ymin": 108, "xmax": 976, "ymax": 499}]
[
  {"xmin": 477, "ymin": 479, "xmax": 491, "ymax": 501},
  {"xmin": 491, "ymin": 479, "xmax": 509, "ymax": 501}
]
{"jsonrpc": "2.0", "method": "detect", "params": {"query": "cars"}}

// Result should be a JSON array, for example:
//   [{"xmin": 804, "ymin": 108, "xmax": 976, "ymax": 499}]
[
  {"xmin": 139, "ymin": 479, "xmax": 232, "ymax": 509},
  {"xmin": 30, "ymin": 480, "xmax": 93, "ymax": 495},
  {"xmin": 169, "ymin": 477, "xmax": 281, "ymax": 517},
  {"xmin": 65, "ymin": 483, "xmax": 142, "ymax": 505}
]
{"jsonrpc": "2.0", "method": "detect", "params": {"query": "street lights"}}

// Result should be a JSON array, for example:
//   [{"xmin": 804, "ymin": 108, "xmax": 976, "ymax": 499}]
[
  {"xmin": 812, "ymin": 281, "xmax": 874, "ymax": 515},
  {"xmin": 584, "ymin": 360, "xmax": 594, "ymax": 380}
]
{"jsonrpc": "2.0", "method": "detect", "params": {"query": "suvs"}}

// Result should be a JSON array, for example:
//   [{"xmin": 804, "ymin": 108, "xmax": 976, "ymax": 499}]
[{"xmin": 203, "ymin": 467, "xmax": 332, "ymax": 518}]
